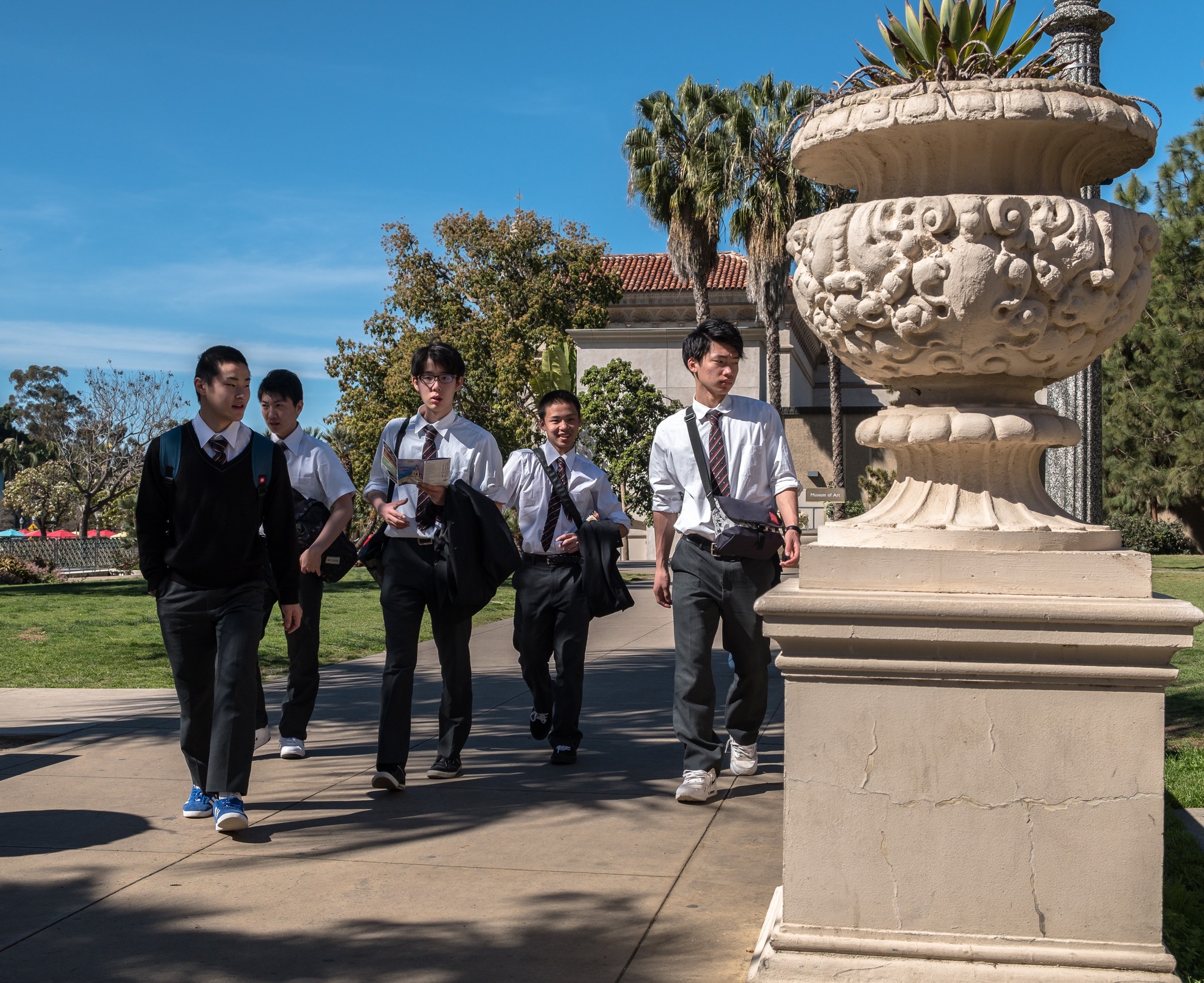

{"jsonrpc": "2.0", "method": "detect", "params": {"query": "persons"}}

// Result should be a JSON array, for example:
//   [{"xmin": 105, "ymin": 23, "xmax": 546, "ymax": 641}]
[
  {"xmin": 501, "ymin": 389, "xmax": 636, "ymax": 765},
  {"xmin": 252, "ymin": 369, "xmax": 358, "ymax": 761},
  {"xmin": 649, "ymin": 315, "xmax": 801, "ymax": 809},
  {"xmin": 360, "ymin": 343, "xmax": 508, "ymax": 796},
  {"xmin": 140, "ymin": 347, "xmax": 307, "ymax": 834}
]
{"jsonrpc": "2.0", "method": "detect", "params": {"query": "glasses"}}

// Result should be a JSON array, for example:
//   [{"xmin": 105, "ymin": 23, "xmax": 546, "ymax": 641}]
[{"xmin": 417, "ymin": 373, "xmax": 457, "ymax": 384}]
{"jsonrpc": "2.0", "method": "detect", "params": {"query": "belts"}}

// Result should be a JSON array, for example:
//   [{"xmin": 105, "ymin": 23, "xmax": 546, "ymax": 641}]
[
  {"xmin": 521, "ymin": 552, "xmax": 584, "ymax": 565},
  {"xmin": 391, "ymin": 537, "xmax": 434, "ymax": 545},
  {"xmin": 682, "ymin": 533, "xmax": 737, "ymax": 557}
]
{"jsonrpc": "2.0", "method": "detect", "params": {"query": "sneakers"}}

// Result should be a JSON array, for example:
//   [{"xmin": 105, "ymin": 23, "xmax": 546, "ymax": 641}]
[
  {"xmin": 529, "ymin": 679, "xmax": 558, "ymax": 740},
  {"xmin": 675, "ymin": 768, "xmax": 718, "ymax": 801},
  {"xmin": 279, "ymin": 732, "xmax": 305, "ymax": 758},
  {"xmin": 213, "ymin": 797, "xmax": 248, "ymax": 831},
  {"xmin": 371, "ymin": 765, "xmax": 406, "ymax": 790},
  {"xmin": 729, "ymin": 734, "xmax": 758, "ymax": 776},
  {"xmin": 550, "ymin": 745, "xmax": 577, "ymax": 763},
  {"xmin": 254, "ymin": 724, "xmax": 271, "ymax": 751},
  {"xmin": 426, "ymin": 753, "xmax": 463, "ymax": 778},
  {"xmin": 183, "ymin": 784, "xmax": 217, "ymax": 817}
]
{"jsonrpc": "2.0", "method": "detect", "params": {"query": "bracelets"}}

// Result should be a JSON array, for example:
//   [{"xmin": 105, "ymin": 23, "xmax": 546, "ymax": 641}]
[{"xmin": 785, "ymin": 524, "xmax": 801, "ymax": 537}]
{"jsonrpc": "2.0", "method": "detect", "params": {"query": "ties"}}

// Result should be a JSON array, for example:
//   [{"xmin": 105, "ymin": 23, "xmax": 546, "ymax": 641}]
[
  {"xmin": 705, "ymin": 411, "xmax": 731, "ymax": 497},
  {"xmin": 276, "ymin": 441, "xmax": 287, "ymax": 452},
  {"xmin": 540, "ymin": 456, "xmax": 568, "ymax": 553},
  {"xmin": 208, "ymin": 438, "xmax": 228, "ymax": 464},
  {"xmin": 415, "ymin": 427, "xmax": 438, "ymax": 530}
]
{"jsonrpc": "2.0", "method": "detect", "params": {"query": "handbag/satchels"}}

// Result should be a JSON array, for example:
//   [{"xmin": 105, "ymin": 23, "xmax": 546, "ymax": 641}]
[
  {"xmin": 358, "ymin": 520, "xmax": 388, "ymax": 590},
  {"xmin": 292, "ymin": 487, "xmax": 358, "ymax": 584},
  {"xmin": 710, "ymin": 495, "xmax": 785, "ymax": 561}
]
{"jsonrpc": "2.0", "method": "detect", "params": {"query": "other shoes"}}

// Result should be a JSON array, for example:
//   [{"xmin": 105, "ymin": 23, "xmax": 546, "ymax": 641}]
[{"xmin": 728, "ymin": 653, "xmax": 735, "ymax": 670}]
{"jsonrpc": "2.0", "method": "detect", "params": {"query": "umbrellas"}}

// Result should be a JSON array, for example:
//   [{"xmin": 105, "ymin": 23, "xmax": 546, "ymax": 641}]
[{"xmin": 0, "ymin": 525, "xmax": 129, "ymax": 539}]
